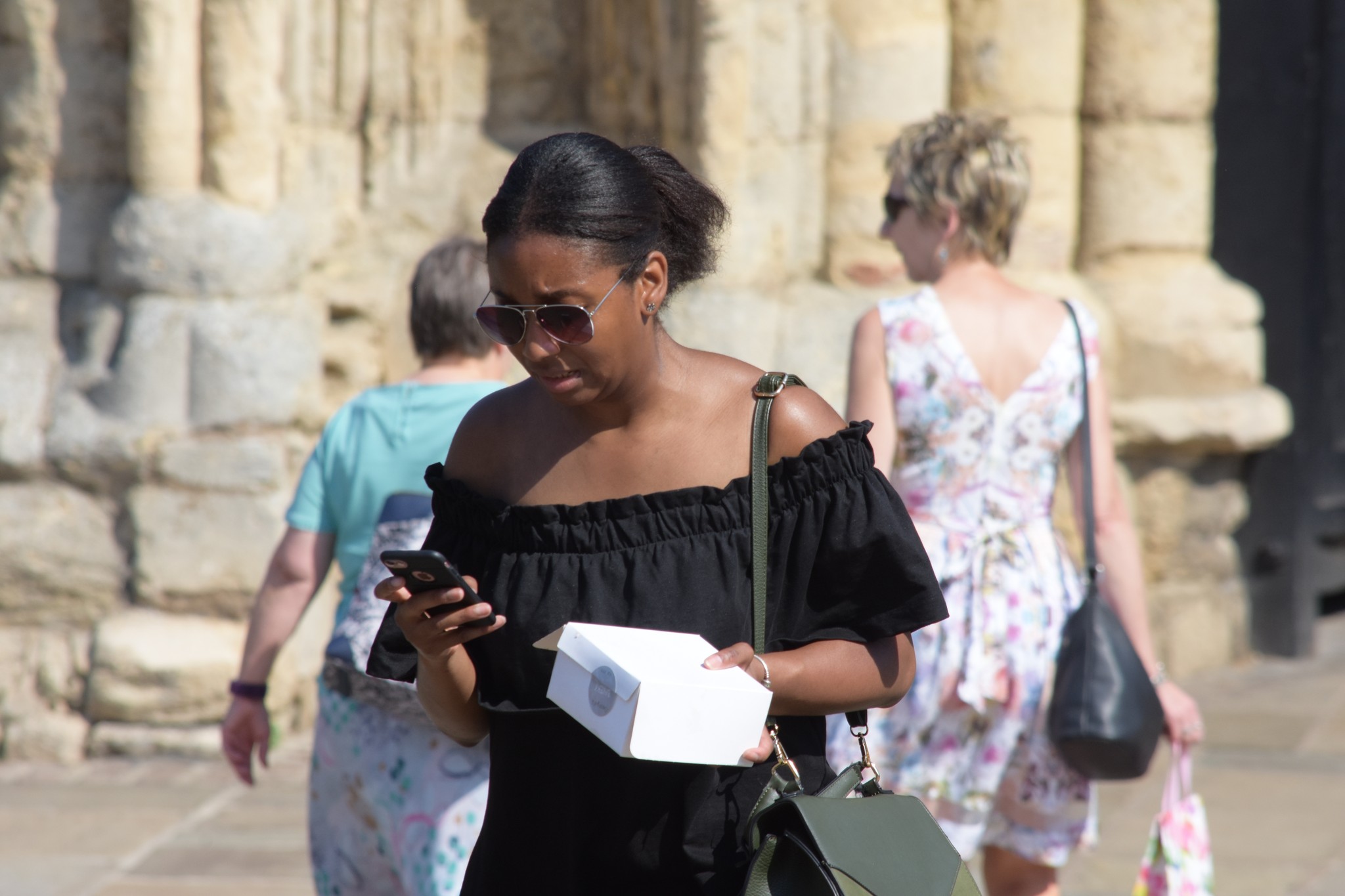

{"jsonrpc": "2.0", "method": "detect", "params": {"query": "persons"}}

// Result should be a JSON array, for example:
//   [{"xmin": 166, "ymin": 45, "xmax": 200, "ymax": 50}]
[
  {"xmin": 222, "ymin": 237, "xmax": 526, "ymax": 896},
  {"xmin": 849, "ymin": 110, "xmax": 1206, "ymax": 896},
  {"xmin": 373, "ymin": 134, "xmax": 950, "ymax": 896}
]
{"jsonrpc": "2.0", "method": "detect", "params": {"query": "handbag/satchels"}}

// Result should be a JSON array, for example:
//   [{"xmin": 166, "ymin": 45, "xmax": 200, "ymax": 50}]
[
  {"xmin": 1130, "ymin": 743, "xmax": 1214, "ymax": 896},
  {"xmin": 733, "ymin": 760, "xmax": 984, "ymax": 896},
  {"xmin": 1047, "ymin": 589, "xmax": 1164, "ymax": 780}
]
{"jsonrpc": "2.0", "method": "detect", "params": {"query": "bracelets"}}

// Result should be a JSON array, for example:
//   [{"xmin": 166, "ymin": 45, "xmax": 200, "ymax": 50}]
[
  {"xmin": 230, "ymin": 677, "xmax": 267, "ymax": 702},
  {"xmin": 754, "ymin": 655, "xmax": 771, "ymax": 693},
  {"xmin": 1151, "ymin": 674, "xmax": 1166, "ymax": 686}
]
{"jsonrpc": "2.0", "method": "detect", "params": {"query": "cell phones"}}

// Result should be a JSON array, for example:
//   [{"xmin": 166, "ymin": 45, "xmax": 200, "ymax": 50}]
[{"xmin": 378, "ymin": 549, "xmax": 496, "ymax": 629}]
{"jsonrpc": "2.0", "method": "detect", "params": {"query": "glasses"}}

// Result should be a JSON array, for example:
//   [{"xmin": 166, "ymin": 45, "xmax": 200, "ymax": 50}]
[
  {"xmin": 473, "ymin": 260, "xmax": 638, "ymax": 346},
  {"xmin": 884, "ymin": 195, "xmax": 912, "ymax": 222}
]
